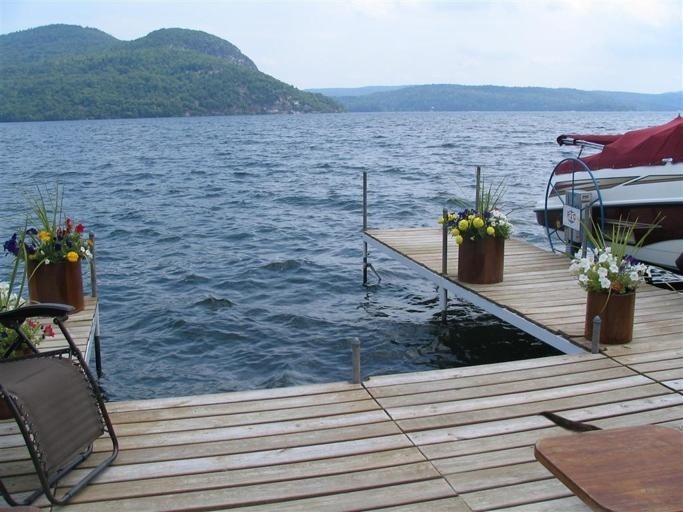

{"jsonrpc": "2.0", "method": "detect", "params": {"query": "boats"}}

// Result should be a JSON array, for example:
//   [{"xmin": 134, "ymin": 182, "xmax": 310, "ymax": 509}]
[{"xmin": 534, "ymin": 116, "xmax": 683, "ymax": 274}]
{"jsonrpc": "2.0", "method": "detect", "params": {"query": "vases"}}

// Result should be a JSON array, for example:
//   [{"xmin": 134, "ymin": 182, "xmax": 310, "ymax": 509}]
[
  {"xmin": 457, "ymin": 234, "xmax": 505, "ymax": 285},
  {"xmin": 585, "ymin": 287, "xmax": 636, "ymax": 343},
  {"xmin": 25, "ymin": 253, "xmax": 84, "ymax": 314}
]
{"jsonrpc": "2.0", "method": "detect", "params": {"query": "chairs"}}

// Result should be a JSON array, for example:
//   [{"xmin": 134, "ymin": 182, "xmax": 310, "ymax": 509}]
[{"xmin": 0, "ymin": 301, "xmax": 118, "ymax": 508}]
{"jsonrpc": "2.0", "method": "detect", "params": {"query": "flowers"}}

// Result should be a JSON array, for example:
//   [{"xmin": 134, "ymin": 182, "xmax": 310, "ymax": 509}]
[
  {"xmin": 1, "ymin": 174, "xmax": 94, "ymax": 266},
  {"xmin": 566, "ymin": 205, "xmax": 678, "ymax": 314},
  {"xmin": 434, "ymin": 173, "xmax": 535, "ymax": 247},
  {"xmin": 1, "ymin": 282, "xmax": 56, "ymax": 364}
]
